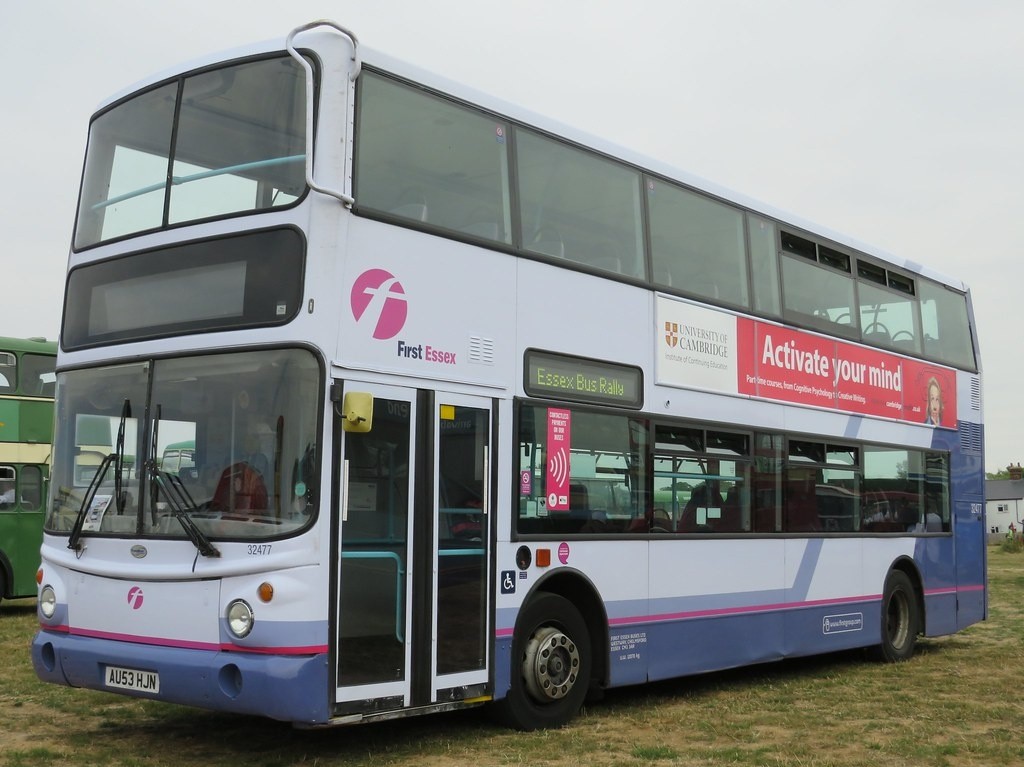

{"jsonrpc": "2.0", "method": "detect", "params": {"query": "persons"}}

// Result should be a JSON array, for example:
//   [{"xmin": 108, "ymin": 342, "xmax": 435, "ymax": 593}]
[
  {"xmin": 211, "ymin": 421, "xmax": 308, "ymax": 522},
  {"xmin": 1006, "ymin": 525, "xmax": 1014, "ymax": 540}
]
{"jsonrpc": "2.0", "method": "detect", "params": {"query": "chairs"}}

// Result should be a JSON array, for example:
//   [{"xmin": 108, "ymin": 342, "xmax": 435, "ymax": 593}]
[
  {"xmin": 677, "ymin": 480, "xmax": 824, "ymax": 533},
  {"xmin": 398, "ymin": 196, "xmax": 940, "ymax": 359},
  {"xmin": 551, "ymin": 484, "xmax": 608, "ymax": 519}
]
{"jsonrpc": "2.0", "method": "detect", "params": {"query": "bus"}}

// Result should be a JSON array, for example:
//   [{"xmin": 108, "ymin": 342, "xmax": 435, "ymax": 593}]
[
  {"xmin": 527, "ymin": 465, "xmax": 924, "ymax": 530},
  {"xmin": 0, "ymin": 335, "xmax": 112, "ymax": 610},
  {"xmin": 115, "ymin": 437, "xmax": 200, "ymax": 518},
  {"xmin": 31, "ymin": 15, "xmax": 986, "ymax": 730}
]
